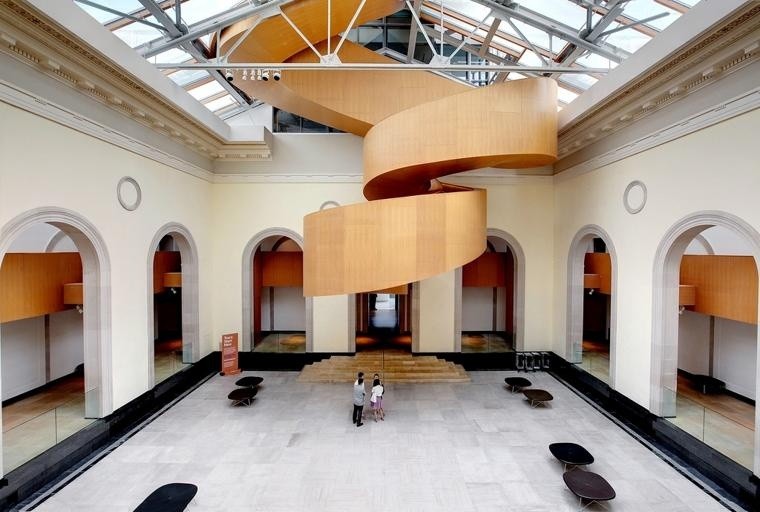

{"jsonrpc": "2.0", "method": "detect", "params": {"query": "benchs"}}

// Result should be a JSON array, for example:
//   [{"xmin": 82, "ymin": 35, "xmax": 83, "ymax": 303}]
[
  {"xmin": 132, "ymin": 483, "xmax": 197, "ymax": 511},
  {"xmin": 505, "ymin": 377, "xmax": 553, "ymax": 408},
  {"xmin": 228, "ymin": 376, "xmax": 264, "ymax": 407},
  {"xmin": 549, "ymin": 443, "xmax": 616, "ymax": 512}
]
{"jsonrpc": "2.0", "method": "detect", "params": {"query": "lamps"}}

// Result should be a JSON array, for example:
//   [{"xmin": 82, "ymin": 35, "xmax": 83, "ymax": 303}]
[{"xmin": 224, "ymin": 68, "xmax": 281, "ymax": 81}]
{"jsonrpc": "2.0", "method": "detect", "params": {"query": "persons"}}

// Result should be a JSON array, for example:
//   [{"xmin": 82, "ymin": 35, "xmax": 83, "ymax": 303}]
[
  {"xmin": 371, "ymin": 379, "xmax": 384, "ymax": 423},
  {"xmin": 372, "ymin": 374, "xmax": 385, "ymax": 416},
  {"xmin": 354, "ymin": 373, "xmax": 366, "ymax": 416},
  {"xmin": 352, "ymin": 377, "xmax": 366, "ymax": 426}
]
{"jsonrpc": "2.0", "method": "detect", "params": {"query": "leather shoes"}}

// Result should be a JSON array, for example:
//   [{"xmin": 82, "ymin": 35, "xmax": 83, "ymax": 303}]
[{"xmin": 353, "ymin": 420, "xmax": 363, "ymax": 426}]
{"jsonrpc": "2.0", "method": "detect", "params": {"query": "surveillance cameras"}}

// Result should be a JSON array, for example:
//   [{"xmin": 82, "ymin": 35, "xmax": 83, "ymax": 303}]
[
  {"xmin": 225, "ymin": 73, "xmax": 233, "ymax": 82},
  {"xmin": 262, "ymin": 73, "xmax": 269, "ymax": 81},
  {"xmin": 273, "ymin": 73, "xmax": 280, "ymax": 81}
]
